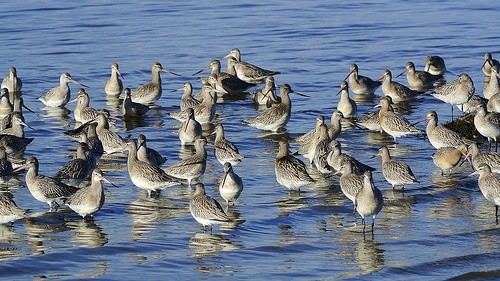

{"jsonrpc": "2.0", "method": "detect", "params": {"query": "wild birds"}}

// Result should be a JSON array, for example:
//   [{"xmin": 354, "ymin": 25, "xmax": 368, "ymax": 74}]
[{"xmin": 0, "ymin": 48, "xmax": 500, "ymax": 234}]
